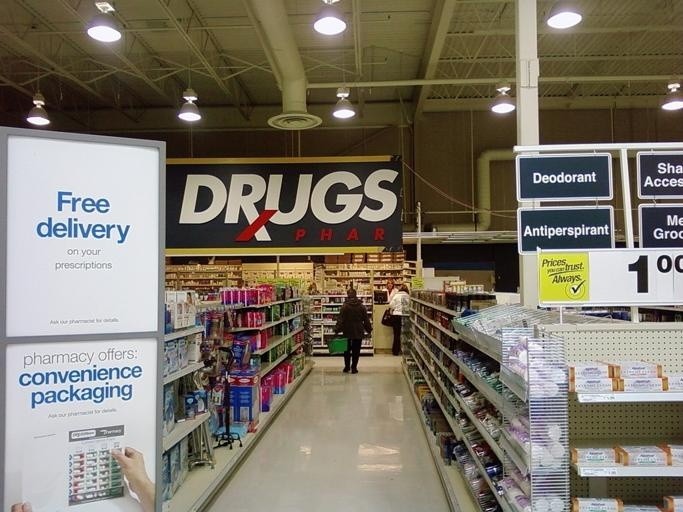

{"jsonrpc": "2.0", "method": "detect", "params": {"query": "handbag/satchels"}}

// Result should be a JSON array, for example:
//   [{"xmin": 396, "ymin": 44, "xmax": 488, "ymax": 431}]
[{"xmin": 381, "ymin": 309, "xmax": 393, "ymax": 327}]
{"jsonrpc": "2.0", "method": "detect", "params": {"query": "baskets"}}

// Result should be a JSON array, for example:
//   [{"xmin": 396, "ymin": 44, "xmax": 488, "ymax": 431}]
[{"xmin": 327, "ymin": 334, "xmax": 348, "ymax": 354}]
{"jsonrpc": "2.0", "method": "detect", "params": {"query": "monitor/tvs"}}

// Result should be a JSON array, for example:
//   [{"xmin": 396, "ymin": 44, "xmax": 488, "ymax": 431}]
[{"xmin": 373, "ymin": 290, "xmax": 389, "ymax": 303}]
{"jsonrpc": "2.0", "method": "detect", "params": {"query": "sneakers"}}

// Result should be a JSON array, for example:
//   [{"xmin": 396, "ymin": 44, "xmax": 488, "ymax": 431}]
[
  {"xmin": 352, "ymin": 369, "xmax": 358, "ymax": 374},
  {"xmin": 343, "ymin": 365, "xmax": 350, "ymax": 372}
]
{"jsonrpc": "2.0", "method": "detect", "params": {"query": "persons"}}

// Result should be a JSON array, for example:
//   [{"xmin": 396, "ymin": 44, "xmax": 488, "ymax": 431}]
[
  {"xmin": 12, "ymin": 447, "xmax": 155, "ymax": 512},
  {"xmin": 389, "ymin": 284, "xmax": 410, "ymax": 355},
  {"xmin": 334, "ymin": 288, "xmax": 372, "ymax": 375},
  {"xmin": 382, "ymin": 279, "xmax": 398, "ymax": 304}
]
